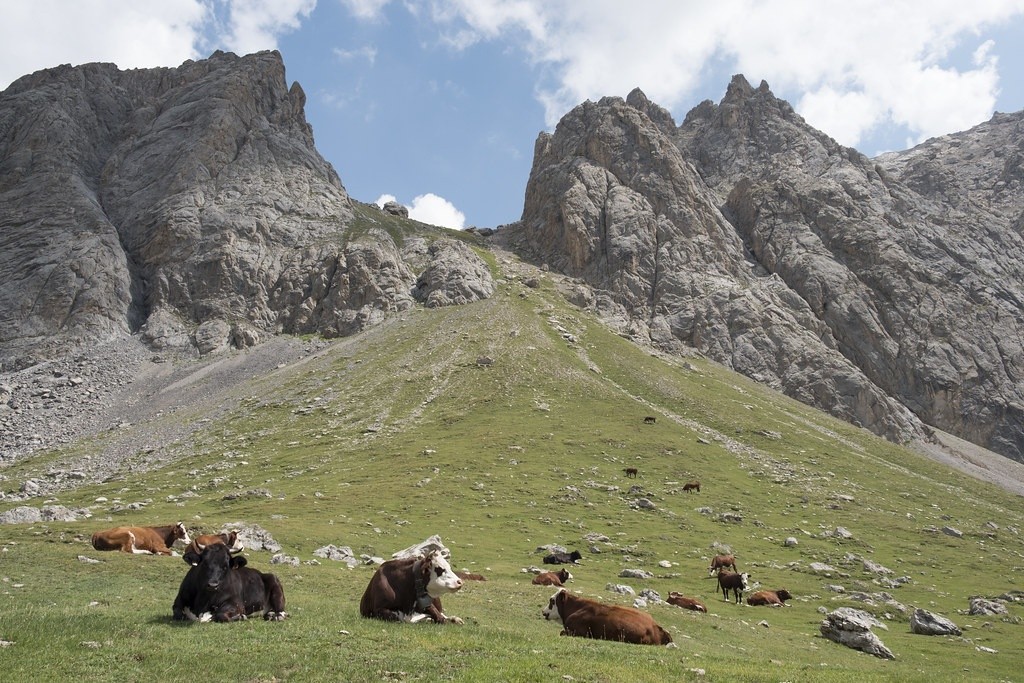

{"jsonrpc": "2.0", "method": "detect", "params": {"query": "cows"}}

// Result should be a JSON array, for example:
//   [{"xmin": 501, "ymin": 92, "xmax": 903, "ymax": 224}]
[
  {"xmin": 543, "ymin": 549, "xmax": 585, "ymax": 565},
  {"xmin": 644, "ymin": 416, "xmax": 656, "ymax": 424},
  {"xmin": 622, "ymin": 468, "xmax": 638, "ymax": 479},
  {"xmin": 360, "ymin": 549, "xmax": 466, "ymax": 625},
  {"xmin": 185, "ymin": 530, "xmax": 242, "ymax": 563},
  {"xmin": 666, "ymin": 590, "xmax": 707, "ymax": 613},
  {"xmin": 542, "ymin": 589, "xmax": 673, "ymax": 645},
  {"xmin": 707, "ymin": 555, "xmax": 737, "ymax": 576},
  {"xmin": 682, "ymin": 481, "xmax": 700, "ymax": 493},
  {"xmin": 92, "ymin": 522, "xmax": 192, "ymax": 558},
  {"xmin": 747, "ymin": 589, "xmax": 793, "ymax": 607},
  {"xmin": 718, "ymin": 571, "xmax": 751, "ymax": 604},
  {"xmin": 532, "ymin": 568, "xmax": 574, "ymax": 588},
  {"xmin": 172, "ymin": 538, "xmax": 290, "ymax": 623}
]
